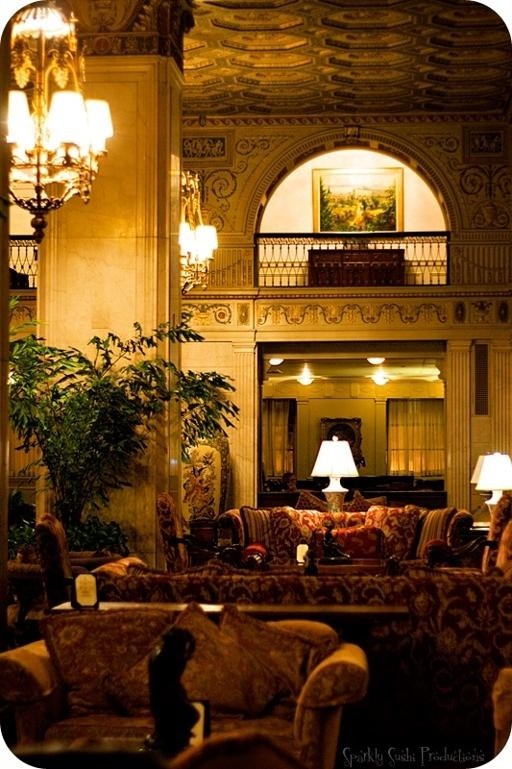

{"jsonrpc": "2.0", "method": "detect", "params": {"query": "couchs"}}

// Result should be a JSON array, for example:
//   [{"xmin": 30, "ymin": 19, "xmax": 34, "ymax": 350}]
[
  {"xmin": 1, "ymin": 620, "xmax": 369, "ymax": 768},
  {"xmin": 170, "ymin": 505, "xmax": 477, "ymax": 579}
]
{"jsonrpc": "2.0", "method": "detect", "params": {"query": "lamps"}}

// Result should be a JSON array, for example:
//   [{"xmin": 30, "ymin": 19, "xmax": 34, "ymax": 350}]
[
  {"xmin": 309, "ymin": 435, "xmax": 359, "ymax": 512},
  {"xmin": 6, "ymin": 1, "xmax": 115, "ymax": 243},
  {"xmin": 471, "ymin": 451, "xmax": 512, "ymax": 527}
]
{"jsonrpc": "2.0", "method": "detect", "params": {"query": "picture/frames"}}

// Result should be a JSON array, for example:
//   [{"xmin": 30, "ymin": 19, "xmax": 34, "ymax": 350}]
[{"xmin": 312, "ymin": 166, "xmax": 405, "ymax": 234}]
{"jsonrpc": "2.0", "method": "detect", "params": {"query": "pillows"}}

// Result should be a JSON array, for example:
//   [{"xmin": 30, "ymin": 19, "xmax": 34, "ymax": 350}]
[
  {"xmin": 234, "ymin": 506, "xmax": 421, "ymax": 560},
  {"xmin": 36, "ymin": 595, "xmax": 328, "ymax": 725}
]
